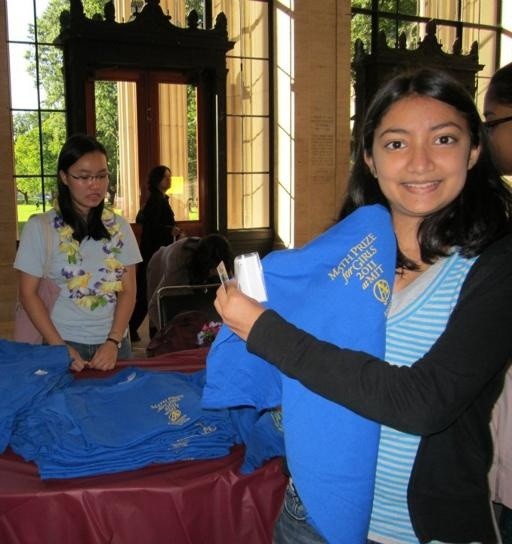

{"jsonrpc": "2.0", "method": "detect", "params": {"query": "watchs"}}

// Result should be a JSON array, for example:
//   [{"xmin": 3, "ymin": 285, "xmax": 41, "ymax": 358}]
[{"xmin": 105, "ymin": 338, "xmax": 125, "ymax": 348}]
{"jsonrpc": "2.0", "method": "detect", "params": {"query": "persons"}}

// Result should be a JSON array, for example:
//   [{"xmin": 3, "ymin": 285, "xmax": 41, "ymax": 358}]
[
  {"xmin": 212, "ymin": 65, "xmax": 512, "ymax": 544},
  {"xmin": 142, "ymin": 229, "xmax": 231, "ymax": 339},
  {"xmin": 128, "ymin": 163, "xmax": 185, "ymax": 341},
  {"xmin": 16, "ymin": 134, "xmax": 145, "ymax": 373},
  {"xmin": 478, "ymin": 63, "xmax": 511, "ymax": 544}
]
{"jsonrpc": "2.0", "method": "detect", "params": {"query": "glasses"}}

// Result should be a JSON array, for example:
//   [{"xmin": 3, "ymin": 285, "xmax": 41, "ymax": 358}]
[{"xmin": 63, "ymin": 170, "xmax": 110, "ymax": 185}]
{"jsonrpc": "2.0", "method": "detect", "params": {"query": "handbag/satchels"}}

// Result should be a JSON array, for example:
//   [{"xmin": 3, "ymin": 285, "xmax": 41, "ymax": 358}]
[{"xmin": 14, "ymin": 273, "xmax": 59, "ymax": 347}]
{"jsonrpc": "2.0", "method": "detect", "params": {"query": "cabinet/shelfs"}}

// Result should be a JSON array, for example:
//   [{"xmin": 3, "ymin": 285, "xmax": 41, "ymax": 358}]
[{"xmin": 0, "ymin": 346, "xmax": 288, "ymax": 544}]
